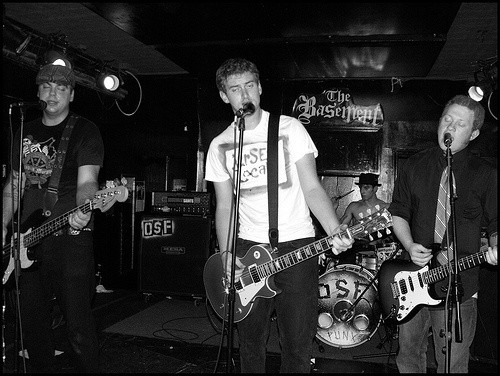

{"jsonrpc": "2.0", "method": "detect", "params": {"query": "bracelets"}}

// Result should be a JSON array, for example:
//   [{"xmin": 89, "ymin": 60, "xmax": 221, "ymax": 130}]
[{"xmin": 220, "ymin": 250, "xmax": 232, "ymax": 255}]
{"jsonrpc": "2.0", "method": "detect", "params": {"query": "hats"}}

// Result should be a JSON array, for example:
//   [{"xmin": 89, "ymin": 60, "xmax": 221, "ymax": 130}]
[
  {"xmin": 37, "ymin": 63, "xmax": 75, "ymax": 88},
  {"xmin": 353, "ymin": 173, "xmax": 380, "ymax": 188}
]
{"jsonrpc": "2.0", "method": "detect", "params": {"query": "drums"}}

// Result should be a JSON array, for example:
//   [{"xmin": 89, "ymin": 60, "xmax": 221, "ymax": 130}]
[
  {"xmin": 315, "ymin": 263, "xmax": 382, "ymax": 349},
  {"xmin": 355, "ymin": 250, "xmax": 377, "ymax": 274}
]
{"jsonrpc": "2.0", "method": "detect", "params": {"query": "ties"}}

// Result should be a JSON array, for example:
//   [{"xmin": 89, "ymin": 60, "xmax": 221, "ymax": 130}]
[{"xmin": 434, "ymin": 164, "xmax": 457, "ymax": 265}]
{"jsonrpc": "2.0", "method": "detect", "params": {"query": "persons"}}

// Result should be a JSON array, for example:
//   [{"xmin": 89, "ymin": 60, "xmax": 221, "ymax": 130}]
[
  {"xmin": 0, "ymin": 65, "xmax": 108, "ymax": 376},
  {"xmin": 337, "ymin": 172, "xmax": 392, "ymax": 235},
  {"xmin": 204, "ymin": 58, "xmax": 356, "ymax": 373},
  {"xmin": 387, "ymin": 94, "xmax": 498, "ymax": 373}
]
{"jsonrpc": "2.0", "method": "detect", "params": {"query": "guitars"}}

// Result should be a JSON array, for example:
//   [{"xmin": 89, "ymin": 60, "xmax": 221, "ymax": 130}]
[
  {"xmin": 0, "ymin": 177, "xmax": 129, "ymax": 284},
  {"xmin": 377, "ymin": 246, "xmax": 500, "ymax": 325},
  {"xmin": 204, "ymin": 205, "xmax": 394, "ymax": 323}
]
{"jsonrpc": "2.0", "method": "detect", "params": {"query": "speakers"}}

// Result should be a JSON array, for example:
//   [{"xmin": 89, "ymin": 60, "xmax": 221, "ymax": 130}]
[{"xmin": 138, "ymin": 215, "xmax": 217, "ymax": 301}]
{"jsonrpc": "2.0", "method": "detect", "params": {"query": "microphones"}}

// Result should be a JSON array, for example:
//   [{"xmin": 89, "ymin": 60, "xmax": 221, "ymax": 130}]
[
  {"xmin": 235, "ymin": 103, "xmax": 256, "ymax": 118},
  {"xmin": 11, "ymin": 100, "xmax": 47, "ymax": 110},
  {"xmin": 443, "ymin": 132, "xmax": 452, "ymax": 146}
]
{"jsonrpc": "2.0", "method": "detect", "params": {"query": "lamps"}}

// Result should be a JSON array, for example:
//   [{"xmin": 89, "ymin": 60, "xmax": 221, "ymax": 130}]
[
  {"xmin": 46, "ymin": 50, "xmax": 72, "ymax": 70},
  {"xmin": 98, "ymin": 73, "xmax": 120, "ymax": 92},
  {"xmin": 468, "ymin": 75, "xmax": 495, "ymax": 103}
]
